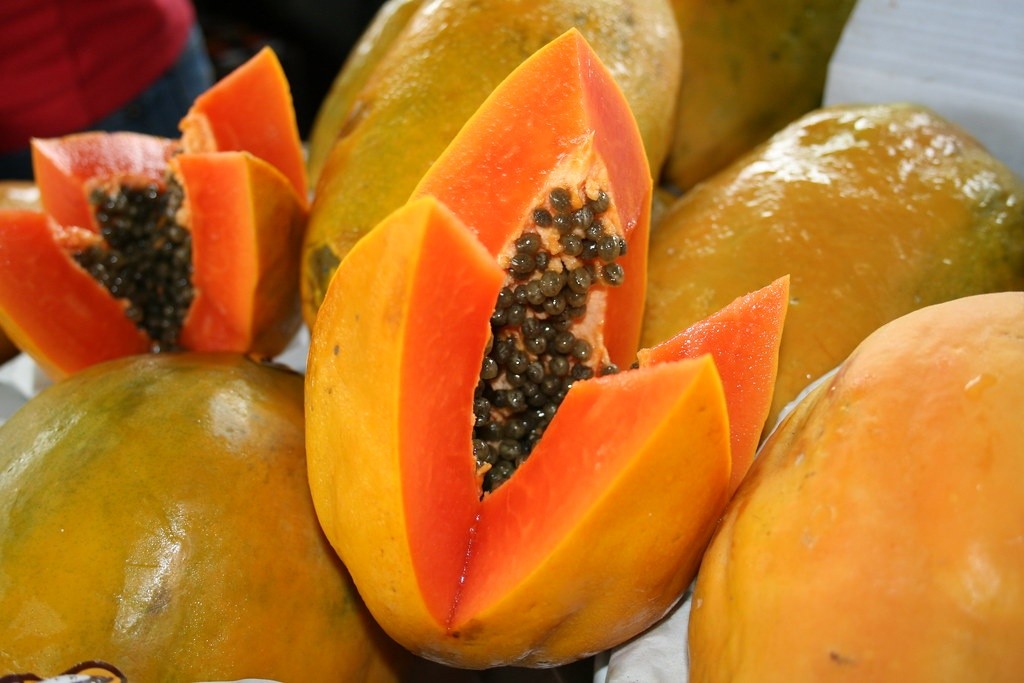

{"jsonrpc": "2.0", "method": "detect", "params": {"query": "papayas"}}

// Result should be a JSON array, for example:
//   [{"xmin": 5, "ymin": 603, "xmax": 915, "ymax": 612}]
[{"xmin": 0, "ymin": 0, "xmax": 1024, "ymax": 683}]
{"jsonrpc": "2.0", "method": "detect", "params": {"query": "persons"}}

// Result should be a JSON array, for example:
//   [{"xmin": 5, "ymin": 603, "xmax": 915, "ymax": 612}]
[{"xmin": 0, "ymin": 0, "xmax": 212, "ymax": 182}]
